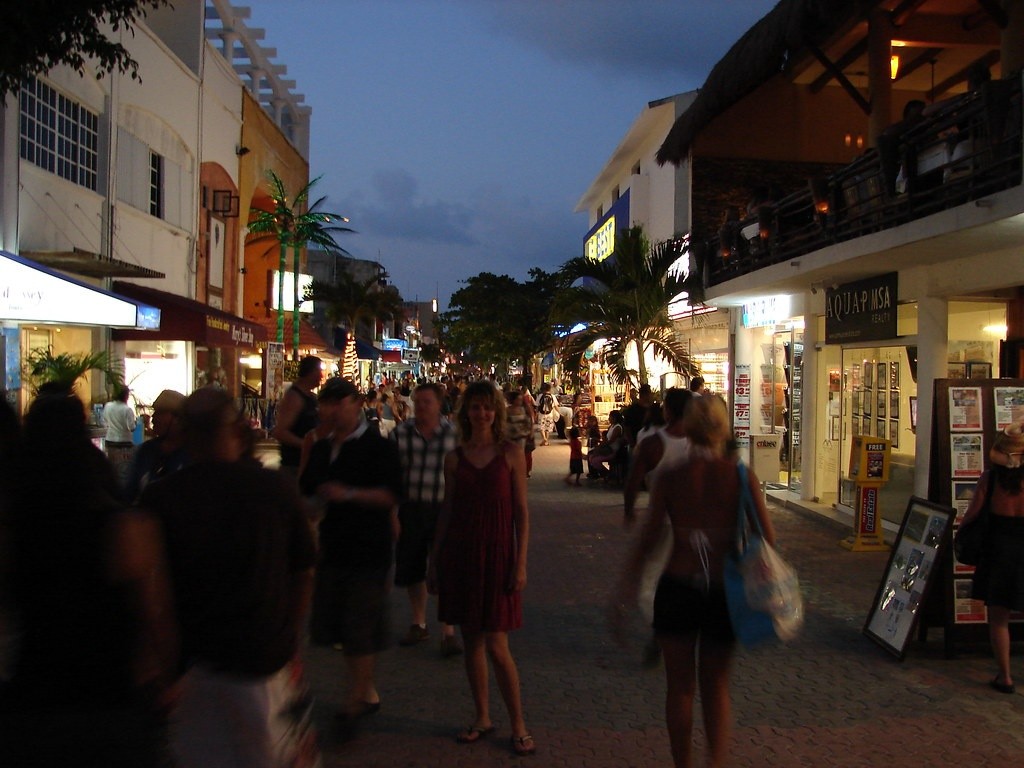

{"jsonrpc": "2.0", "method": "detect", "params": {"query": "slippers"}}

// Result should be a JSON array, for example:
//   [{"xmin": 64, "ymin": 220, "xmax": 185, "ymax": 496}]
[
  {"xmin": 511, "ymin": 735, "xmax": 536, "ymax": 756},
  {"xmin": 456, "ymin": 726, "xmax": 495, "ymax": 744},
  {"xmin": 991, "ymin": 674, "xmax": 1015, "ymax": 693}
]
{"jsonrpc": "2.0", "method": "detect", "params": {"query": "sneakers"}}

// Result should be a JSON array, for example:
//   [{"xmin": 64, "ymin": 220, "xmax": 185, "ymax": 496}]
[
  {"xmin": 399, "ymin": 624, "xmax": 431, "ymax": 646},
  {"xmin": 440, "ymin": 636, "xmax": 462, "ymax": 654}
]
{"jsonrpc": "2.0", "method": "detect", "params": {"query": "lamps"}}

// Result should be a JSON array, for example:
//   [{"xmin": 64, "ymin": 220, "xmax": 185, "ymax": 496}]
[{"xmin": 235, "ymin": 147, "xmax": 249, "ymax": 157}]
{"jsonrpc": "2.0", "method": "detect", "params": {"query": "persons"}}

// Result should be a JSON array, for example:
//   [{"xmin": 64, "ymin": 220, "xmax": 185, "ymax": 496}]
[
  {"xmin": 453, "ymin": 369, "xmax": 564, "ymax": 478},
  {"xmin": 632, "ymin": 393, "xmax": 776, "ymax": 768},
  {"xmin": 565, "ymin": 377, "xmax": 705, "ymax": 525},
  {"xmin": 960, "ymin": 419, "xmax": 1024, "ymax": 693},
  {"xmin": 428, "ymin": 380, "xmax": 536, "ymax": 756},
  {"xmin": 271, "ymin": 356, "xmax": 461, "ymax": 718},
  {"xmin": 0, "ymin": 380, "xmax": 316, "ymax": 768}
]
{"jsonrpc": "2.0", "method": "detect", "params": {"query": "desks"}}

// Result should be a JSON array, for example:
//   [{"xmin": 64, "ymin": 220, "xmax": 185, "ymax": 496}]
[{"xmin": 893, "ymin": 141, "xmax": 951, "ymax": 194}]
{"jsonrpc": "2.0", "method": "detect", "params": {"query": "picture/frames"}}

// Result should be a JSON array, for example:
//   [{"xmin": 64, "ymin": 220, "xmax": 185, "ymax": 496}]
[
  {"xmin": 852, "ymin": 362, "xmax": 899, "ymax": 448},
  {"xmin": 945, "ymin": 362, "xmax": 968, "ymax": 378},
  {"xmin": 969, "ymin": 362, "xmax": 993, "ymax": 379},
  {"xmin": 862, "ymin": 494, "xmax": 957, "ymax": 661}
]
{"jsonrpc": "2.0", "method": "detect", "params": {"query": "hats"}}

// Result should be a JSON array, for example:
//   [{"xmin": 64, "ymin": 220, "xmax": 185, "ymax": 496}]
[
  {"xmin": 318, "ymin": 378, "xmax": 360, "ymax": 402},
  {"xmin": 153, "ymin": 390, "xmax": 186, "ymax": 416},
  {"xmin": 989, "ymin": 421, "xmax": 1024, "ymax": 467}
]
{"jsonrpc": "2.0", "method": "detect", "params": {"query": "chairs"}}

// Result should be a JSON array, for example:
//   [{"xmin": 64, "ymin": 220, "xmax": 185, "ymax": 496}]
[
  {"xmin": 604, "ymin": 440, "xmax": 630, "ymax": 486},
  {"xmin": 941, "ymin": 80, "xmax": 1023, "ymax": 203}
]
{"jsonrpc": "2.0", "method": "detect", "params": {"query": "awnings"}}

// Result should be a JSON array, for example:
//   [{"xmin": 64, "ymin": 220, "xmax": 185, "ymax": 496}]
[
  {"xmin": 0, "ymin": 250, "xmax": 160, "ymax": 332},
  {"xmin": 21, "ymin": 185, "xmax": 165, "ymax": 280},
  {"xmin": 337, "ymin": 327, "xmax": 380, "ymax": 360},
  {"xmin": 110, "ymin": 280, "xmax": 268, "ymax": 350}
]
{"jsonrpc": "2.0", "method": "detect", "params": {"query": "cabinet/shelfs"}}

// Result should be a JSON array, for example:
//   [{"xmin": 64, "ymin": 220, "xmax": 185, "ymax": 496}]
[{"xmin": 590, "ymin": 368, "xmax": 631, "ymax": 432}]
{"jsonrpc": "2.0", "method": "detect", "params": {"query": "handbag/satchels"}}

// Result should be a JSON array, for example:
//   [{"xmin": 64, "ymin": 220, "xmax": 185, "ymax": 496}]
[
  {"xmin": 726, "ymin": 462, "xmax": 803, "ymax": 643},
  {"xmin": 954, "ymin": 468, "xmax": 995, "ymax": 565}
]
